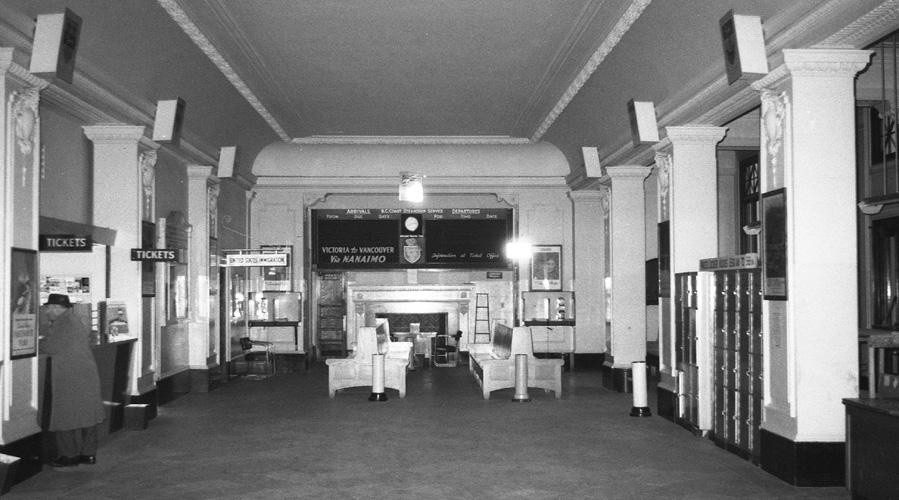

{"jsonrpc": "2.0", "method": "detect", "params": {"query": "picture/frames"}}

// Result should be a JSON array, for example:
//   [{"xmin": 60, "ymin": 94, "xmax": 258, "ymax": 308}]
[
  {"xmin": 9, "ymin": 246, "xmax": 39, "ymax": 361},
  {"xmin": 141, "ymin": 219, "xmax": 157, "ymax": 299},
  {"xmin": 529, "ymin": 243, "xmax": 564, "ymax": 292},
  {"xmin": 259, "ymin": 245, "xmax": 293, "ymax": 293},
  {"xmin": 759, "ymin": 186, "xmax": 788, "ymax": 302}
]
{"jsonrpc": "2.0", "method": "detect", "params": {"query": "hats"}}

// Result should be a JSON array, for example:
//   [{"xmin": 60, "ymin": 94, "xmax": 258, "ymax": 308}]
[{"xmin": 43, "ymin": 294, "xmax": 72, "ymax": 307}]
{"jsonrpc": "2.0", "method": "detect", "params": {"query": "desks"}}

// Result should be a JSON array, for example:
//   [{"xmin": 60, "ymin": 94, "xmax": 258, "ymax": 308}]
[{"xmin": 392, "ymin": 331, "xmax": 436, "ymax": 369}]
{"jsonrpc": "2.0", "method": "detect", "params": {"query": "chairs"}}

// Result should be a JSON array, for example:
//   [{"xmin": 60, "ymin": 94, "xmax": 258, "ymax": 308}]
[
  {"xmin": 239, "ymin": 337, "xmax": 277, "ymax": 380},
  {"xmin": 434, "ymin": 331, "xmax": 462, "ymax": 369}
]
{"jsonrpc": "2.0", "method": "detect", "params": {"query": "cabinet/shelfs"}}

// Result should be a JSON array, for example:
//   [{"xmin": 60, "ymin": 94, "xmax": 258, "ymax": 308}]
[
  {"xmin": 712, "ymin": 270, "xmax": 763, "ymax": 456},
  {"xmin": 673, "ymin": 272, "xmax": 700, "ymax": 431}
]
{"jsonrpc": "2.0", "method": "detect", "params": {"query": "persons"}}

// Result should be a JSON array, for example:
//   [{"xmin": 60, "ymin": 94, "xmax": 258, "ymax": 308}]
[
  {"xmin": 38, "ymin": 294, "xmax": 107, "ymax": 467},
  {"xmin": 534, "ymin": 256, "xmax": 559, "ymax": 279}
]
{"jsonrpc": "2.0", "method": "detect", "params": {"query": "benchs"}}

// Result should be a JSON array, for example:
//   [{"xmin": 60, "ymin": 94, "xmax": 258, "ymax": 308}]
[
  {"xmin": 468, "ymin": 323, "xmax": 568, "ymax": 400},
  {"xmin": 325, "ymin": 319, "xmax": 414, "ymax": 401}
]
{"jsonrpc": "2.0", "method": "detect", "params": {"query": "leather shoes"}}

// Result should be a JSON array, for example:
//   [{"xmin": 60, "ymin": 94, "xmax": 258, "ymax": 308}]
[
  {"xmin": 82, "ymin": 455, "xmax": 96, "ymax": 464},
  {"xmin": 49, "ymin": 456, "xmax": 79, "ymax": 467}
]
